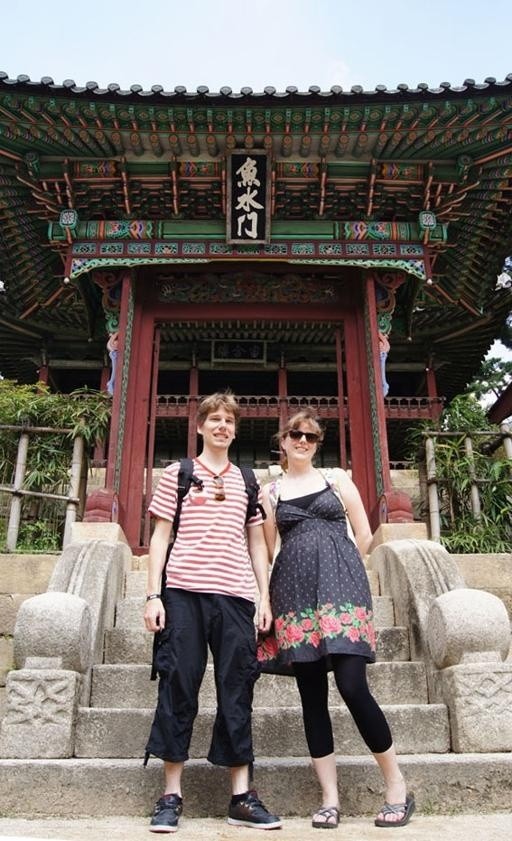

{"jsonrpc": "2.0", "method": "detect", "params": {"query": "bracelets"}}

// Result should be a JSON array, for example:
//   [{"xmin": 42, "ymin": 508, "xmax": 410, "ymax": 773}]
[{"xmin": 145, "ymin": 593, "xmax": 162, "ymax": 601}]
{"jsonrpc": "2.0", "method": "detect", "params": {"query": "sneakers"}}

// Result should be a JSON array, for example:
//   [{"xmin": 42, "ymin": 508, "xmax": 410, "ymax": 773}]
[
  {"xmin": 227, "ymin": 790, "xmax": 284, "ymax": 830},
  {"xmin": 147, "ymin": 794, "xmax": 184, "ymax": 832}
]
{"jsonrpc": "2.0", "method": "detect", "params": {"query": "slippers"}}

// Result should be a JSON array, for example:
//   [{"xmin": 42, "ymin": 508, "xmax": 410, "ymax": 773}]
[
  {"xmin": 373, "ymin": 794, "xmax": 417, "ymax": 828},
  {"xmin": 311, "ymin": 805, "xmax": 341, "ymax": 829}
]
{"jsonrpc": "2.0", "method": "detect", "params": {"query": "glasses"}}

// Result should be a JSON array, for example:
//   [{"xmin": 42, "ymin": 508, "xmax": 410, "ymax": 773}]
[
  {"xmin": 286, "ymin": 429, "xmax": 321, "ymax": 444},
  {"xmin": 212, "ymin": 474, "xmax": 227, "ymax": 502}
]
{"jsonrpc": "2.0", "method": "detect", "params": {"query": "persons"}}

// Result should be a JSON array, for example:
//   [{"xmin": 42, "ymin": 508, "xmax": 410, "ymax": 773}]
[
  {"xmin": 261, "ymin": 405, "xmax": 417, "ymax": 828},
  {"xmin": 143, "ymin": 393, "xmax": 287, "ymax": 832}
]
